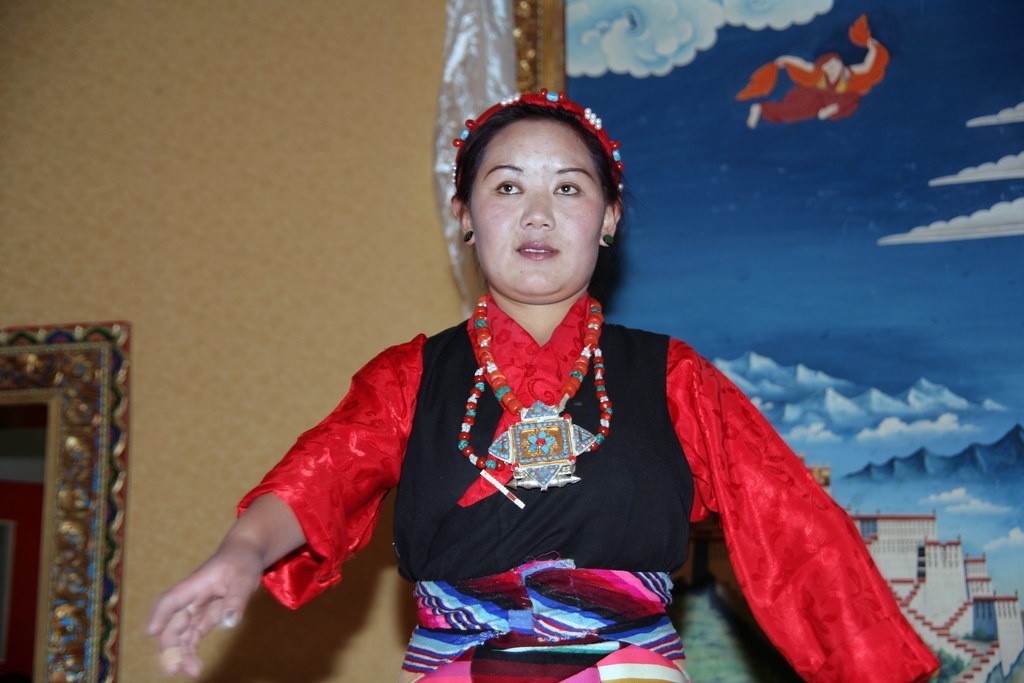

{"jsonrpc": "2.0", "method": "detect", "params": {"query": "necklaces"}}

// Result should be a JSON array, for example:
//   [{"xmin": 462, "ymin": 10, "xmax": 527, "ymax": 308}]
[{"xmin": 457, "ymin": 294, "xmax": 612, "ymax": 508}]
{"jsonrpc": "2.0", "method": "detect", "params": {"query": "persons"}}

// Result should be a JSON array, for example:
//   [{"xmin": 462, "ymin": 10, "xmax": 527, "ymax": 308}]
[{"xmin": 145, "ymin": 87, "xmax": 941, "ymax": 683}]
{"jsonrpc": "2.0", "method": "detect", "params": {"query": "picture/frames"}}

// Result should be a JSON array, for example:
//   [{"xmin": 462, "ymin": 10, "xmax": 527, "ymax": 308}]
[
  {"xmin": 510, "ymin": 1, "xmax": 1024, "ymax": 681},
  {"xmin": 0, "ymin": 321, "xmax": 132, "ymax": 683}
]
